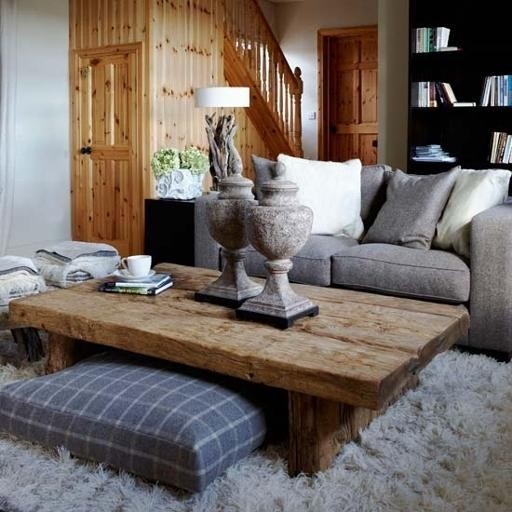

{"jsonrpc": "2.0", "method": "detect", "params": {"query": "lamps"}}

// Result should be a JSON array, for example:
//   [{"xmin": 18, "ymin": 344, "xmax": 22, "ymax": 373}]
[{"xmin": 196, "ymin": 88, "xmax": 250, "ymax": 117}]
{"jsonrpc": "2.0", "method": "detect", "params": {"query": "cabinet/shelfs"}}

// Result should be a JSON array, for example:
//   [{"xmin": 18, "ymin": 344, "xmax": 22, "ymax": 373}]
[{"xmin": 406, "ymin": 0, "xmax": 511, "ymax": 195}]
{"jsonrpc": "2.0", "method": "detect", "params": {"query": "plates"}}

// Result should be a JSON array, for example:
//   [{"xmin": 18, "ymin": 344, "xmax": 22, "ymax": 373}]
[{"xmin": 113, "ymin": 269, "xmax": 156, "ymax": 280}]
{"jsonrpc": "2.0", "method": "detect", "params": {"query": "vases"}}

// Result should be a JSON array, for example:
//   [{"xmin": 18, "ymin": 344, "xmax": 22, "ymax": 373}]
[{"xmin": 154, "ymin": 170, "xmax": 206, "ymax": 201}]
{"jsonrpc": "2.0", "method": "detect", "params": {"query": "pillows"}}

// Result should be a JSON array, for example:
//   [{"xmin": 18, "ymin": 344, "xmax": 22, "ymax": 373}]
[
  {"xmin": 278, "ymin": 154, "xmax": 363, "ymax": 239},
  {"xmin": 253, "ymin": 155, "xmax": 277, "ymax": 200},
  {"xmin": 434, "ymin": 170, "xmax": 512, "ymax": 257},
  {"xmin": 358, "ymin": 164, "xmax": 390, "ymax": 242},
  {"xmin": 362, "ymin": 165, "xmax": 463, "ymax": 250},
  {"xmin": 0, "ymin": 353, "xmax": 272, "ymax": 492}
]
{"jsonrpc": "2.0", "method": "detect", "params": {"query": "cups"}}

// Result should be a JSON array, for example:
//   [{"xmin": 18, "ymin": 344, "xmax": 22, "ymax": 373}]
[{"xmin": 121, "ymin": 255, "xmax": 152, "ymax": 276}]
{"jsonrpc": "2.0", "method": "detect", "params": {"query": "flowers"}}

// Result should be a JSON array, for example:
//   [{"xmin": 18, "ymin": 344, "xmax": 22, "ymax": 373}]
[{"xmin": 152, "ymin": 147, "xmax": 210, "ymax": 171}]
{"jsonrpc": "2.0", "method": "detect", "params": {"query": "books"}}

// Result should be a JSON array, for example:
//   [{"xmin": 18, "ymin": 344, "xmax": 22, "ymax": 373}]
[
  {"xmin": 414, "ymin": 25, "xmax": 462, "ymax": 52},
  {"xmin": 104, "ymin": 273, "xmax": 171, "ymax": 290},
  {"xmin": 453, "ymin": 103, "xmax": 477, "ymax": 108},
  {"xmin": 412, "ymin": 143, "xmax": 456, "ymax": 163},
  {"xmin": 97, "ymin": 280, "xmax": 175, "ymax": 296},
  {"xmin": 488, "ymin": 130, "xmax": 512, "ymax": 164},
  {"xmin": 436, "ymin": 83, "xmax": 457, "ymax": 104},
  {"xmin": 410, "ymin": 80, "xmax": 437, "ymax": 107},
  {"xmin": 480, "ymin": 75, "xmax": 512, "ymax": 107}
]
